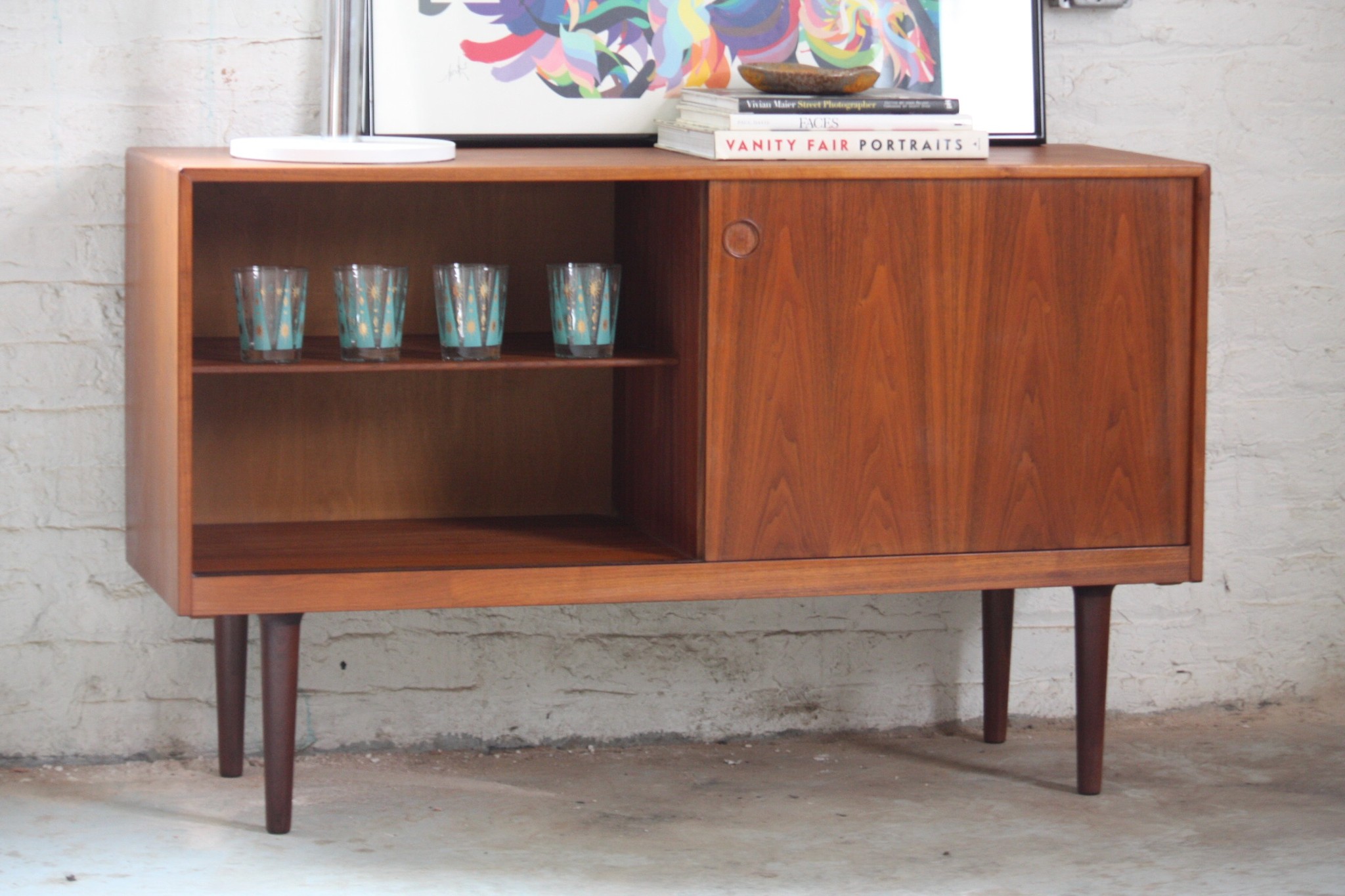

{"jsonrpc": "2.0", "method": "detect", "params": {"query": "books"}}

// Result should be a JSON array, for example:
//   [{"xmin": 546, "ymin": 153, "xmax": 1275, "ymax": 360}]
[{"xmin": 653, "ymin": 86, "xmax": 988, "ymax": 161}]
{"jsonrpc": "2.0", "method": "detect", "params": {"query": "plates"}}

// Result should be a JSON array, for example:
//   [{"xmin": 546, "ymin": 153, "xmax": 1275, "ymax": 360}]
[{"xmin": 733, "ymin": 64, "xmax": 880, "ymax": 93}]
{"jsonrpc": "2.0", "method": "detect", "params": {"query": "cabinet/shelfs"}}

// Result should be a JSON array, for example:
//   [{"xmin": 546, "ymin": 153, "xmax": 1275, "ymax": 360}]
[{"xmin": 126, "ymin": 135, "xmax": 1208, "ymax": 831}]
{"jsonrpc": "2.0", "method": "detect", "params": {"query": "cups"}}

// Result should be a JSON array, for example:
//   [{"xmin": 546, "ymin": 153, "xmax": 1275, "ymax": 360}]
[
  {"xmin": 331, "ymin": 265, "xmax": 409, "ymax": 364},
  {"xmin": 430, "ymin": 264, "xmax": 510, "ymax": 361},
  {"xmin": 233, "ymin": 264, "xmax": 305, "ymax": 364},
  {"xmin": 543, "ymin": 262, "xmax": 624, "ymax": 357}
]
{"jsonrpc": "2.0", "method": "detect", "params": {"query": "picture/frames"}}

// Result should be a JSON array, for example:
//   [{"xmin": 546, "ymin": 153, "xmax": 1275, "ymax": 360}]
[{"xmin": 362, "ymin": 0, "xmax": 1048, "ymax": 148}]
